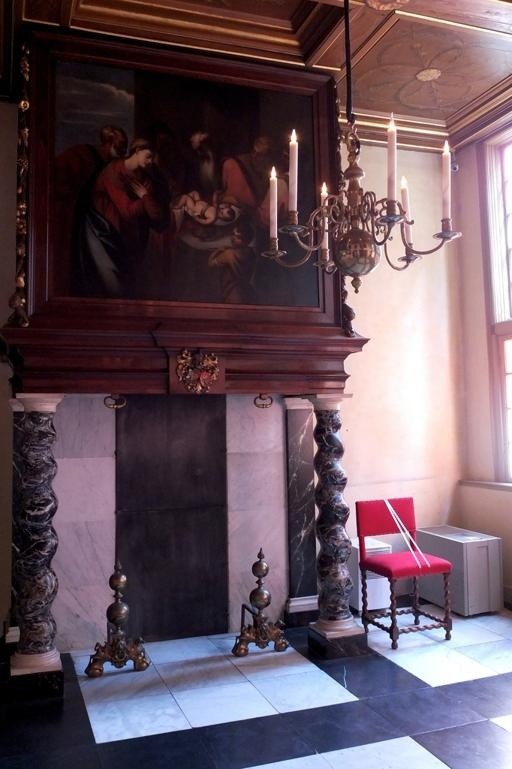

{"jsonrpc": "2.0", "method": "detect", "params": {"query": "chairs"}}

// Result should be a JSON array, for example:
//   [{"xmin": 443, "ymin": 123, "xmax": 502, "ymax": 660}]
[{"xmin": 355, "ymin": 498, "xmax": 451, "ymax": 650}]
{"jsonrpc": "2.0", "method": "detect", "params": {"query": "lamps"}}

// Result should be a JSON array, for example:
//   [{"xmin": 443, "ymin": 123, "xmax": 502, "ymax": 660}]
[{"xmin": 267, "ymin": 0, "xmax": 463, "ymax": 292}]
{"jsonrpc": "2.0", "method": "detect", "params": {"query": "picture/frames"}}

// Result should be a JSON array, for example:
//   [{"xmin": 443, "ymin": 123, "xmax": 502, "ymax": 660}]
[{"xmin": 18, "ymin": 27, "xmax": 342, "ymax": 320}]
